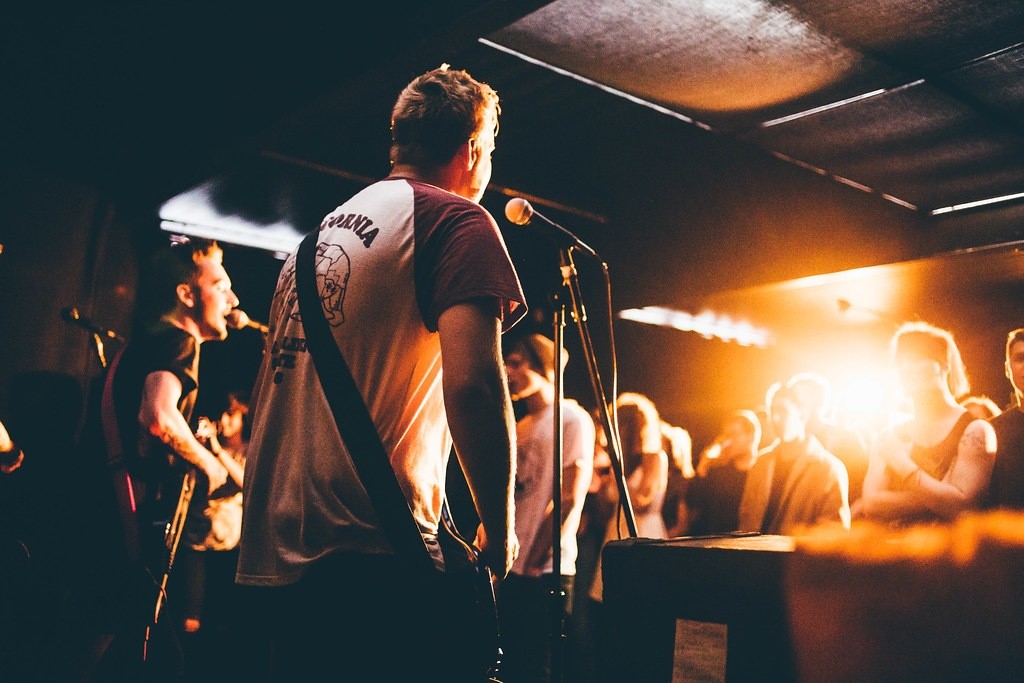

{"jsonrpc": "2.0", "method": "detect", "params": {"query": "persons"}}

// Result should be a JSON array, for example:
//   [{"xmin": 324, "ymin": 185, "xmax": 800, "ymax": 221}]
[
  {"xmin": 63, "ymin": 237, "xmax": 248, "ymax": 683},
  {"xmin": 0, "ymin": 419, "xmax": 25, "ymax": 473},
  {"xmin": 235, "ymin": 69, "xmax": 529, "ymax": 683},
  {"xmin": 505, "ymin": 333, "xmax": 596, "ymax": 683},
  {"xmin": 578, "ymin": 324, "xmax": 1024, "ymax": 683}
]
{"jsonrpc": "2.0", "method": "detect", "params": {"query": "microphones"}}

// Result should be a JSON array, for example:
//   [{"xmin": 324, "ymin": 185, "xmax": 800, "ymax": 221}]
[
  {"xmin": 62, "ymin": 307, "xmax": 125, "ymax": 345},
  {"xmin": 505, "ymin": 197, "xmax": 598, "ymax": 260},
  {"xmin": 227, "ymin": 309, "xmax": 269, "ymax": 334}
]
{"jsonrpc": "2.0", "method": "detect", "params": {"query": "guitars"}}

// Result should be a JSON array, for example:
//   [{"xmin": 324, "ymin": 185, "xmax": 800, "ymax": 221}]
[{"xmin": 146, "ymin": 441, "xmax": 250, "ymax": 554}]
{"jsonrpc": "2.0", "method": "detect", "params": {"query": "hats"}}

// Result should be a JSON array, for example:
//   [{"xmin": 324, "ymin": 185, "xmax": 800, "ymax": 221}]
[{"xmin": 527, "ymin": 333, "xmax": 569, "ymax": 398}]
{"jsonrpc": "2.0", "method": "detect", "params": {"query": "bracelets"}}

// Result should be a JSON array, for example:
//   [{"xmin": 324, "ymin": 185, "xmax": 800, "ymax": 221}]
[{"xmin": 0, "ymin": 441, "xmax": 21, "ymax": 464}]
